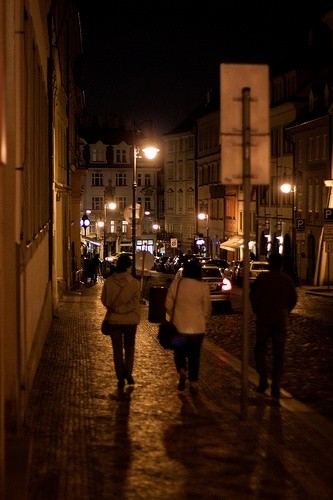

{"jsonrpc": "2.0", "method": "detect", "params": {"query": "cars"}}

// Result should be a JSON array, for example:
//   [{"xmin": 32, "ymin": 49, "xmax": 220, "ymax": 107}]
[
  {"xmin": 165, "ymin": 265, "xmax": 231, "ymax": 308},
  {"xmin": 235, "ymin": 260, "xmax": 269, "ymax": 286},
  {"xmin": 157, "ymin": 253, "xmax": 240, "ymax": 282}
]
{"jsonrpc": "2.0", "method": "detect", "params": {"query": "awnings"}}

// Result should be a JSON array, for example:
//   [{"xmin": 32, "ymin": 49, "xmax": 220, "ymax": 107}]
[
  {"xmin": 80, "ymin": 234, "xmax": 101, "ymax": 246},
  {"xmin": 219, "ymin": 235, "xmax": 256, "ymax": 253}
]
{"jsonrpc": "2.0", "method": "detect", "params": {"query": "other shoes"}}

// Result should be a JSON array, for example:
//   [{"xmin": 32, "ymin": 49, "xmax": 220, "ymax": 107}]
[
  {"xmin": 269, "ymin": 384, "xmax": 281, "ymax": 397},
  {"xmin": 257, "ymin": 381, "xmax": 266, "ymax": 392},
  {"xmin": 124, "ymin": 375, "xmax": 135, "ymax": 385},
  {"xmin": 189, "ymin": 386, "xmax": 198, "ymax": 396},
  {"xmin": 177, "ymin": 374, "xmax": 186, "ymax": 391},
  {"xmin": 118, "ymin": 375, "xmax": 125, "ymax": 389}
]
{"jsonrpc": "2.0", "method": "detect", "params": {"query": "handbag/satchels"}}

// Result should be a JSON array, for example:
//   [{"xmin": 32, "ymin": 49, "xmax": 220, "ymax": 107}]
[
  {"xmin": 156, "ymin": 321, "xmax": 179, "ymax": 350},
  {"xmin": 100, "ymin": 316, "xmax": 113, "ymax": 336}
]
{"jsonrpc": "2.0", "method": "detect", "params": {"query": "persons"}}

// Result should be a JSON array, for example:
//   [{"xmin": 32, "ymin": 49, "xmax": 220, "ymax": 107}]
[
  {"xmin": 250, "ymin": 253, "xmax": 297, "ymax": 399},
  {"xmin": 81, "ymin": 232, "xmax": 278, "ymax": 285},
  {"xmin": 101, "ymin": 254, "xmax": 142, "ymax": 389},
  {"xmin": 165, "ymin": 261, "xmax": 211, "ymax": 393}
]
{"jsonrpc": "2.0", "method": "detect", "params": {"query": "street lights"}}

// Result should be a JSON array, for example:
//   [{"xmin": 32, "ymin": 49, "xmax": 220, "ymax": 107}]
[
  {"xmin": 198, "ymin": 213, "xmax": 211, "ymax": 258},
  {"xmin": 281, "ymin": 183, "xmax": 299, "ymax": 286},
  {"xmin": 130, "ymin": 119, "xmax": 160, "ymax": 278},
  {"xmin": 102, "ymin": 202, "xmax": 117, "ymax": 279}
]
